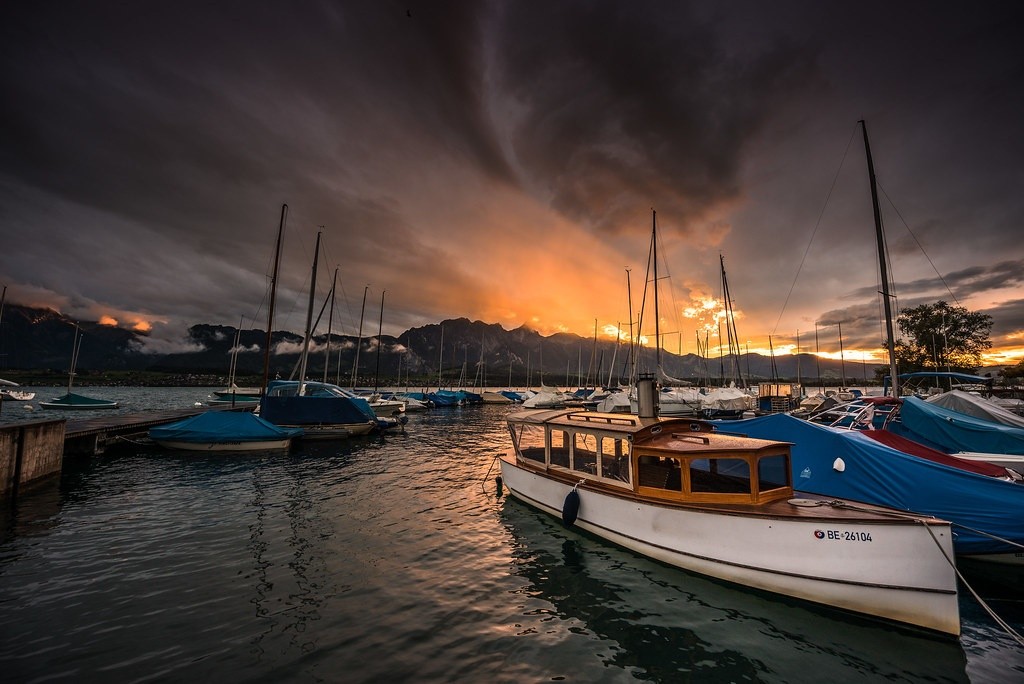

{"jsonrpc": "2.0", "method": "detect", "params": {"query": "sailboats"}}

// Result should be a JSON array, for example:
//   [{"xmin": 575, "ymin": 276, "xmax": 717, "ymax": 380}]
[{"xmin": 204, "ymin": 119, "xmax": 1024, "ymax": 562}]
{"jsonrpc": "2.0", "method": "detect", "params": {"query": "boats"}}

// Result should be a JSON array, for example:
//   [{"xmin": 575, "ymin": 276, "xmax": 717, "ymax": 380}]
[
  {"xmin": 38, "ymin": 320, "xmax": 119, "ymax": 409},
  {"xmin": 149, "ymin": 410, "xmax": 307, "ymax": 451},
  {"xmin": 496, "ymin": 372, "xmax": 963, "ymax": 640},
  {"xmin": 0, "ymin": 389, "xmax": 36, "ymax": 401}
]
